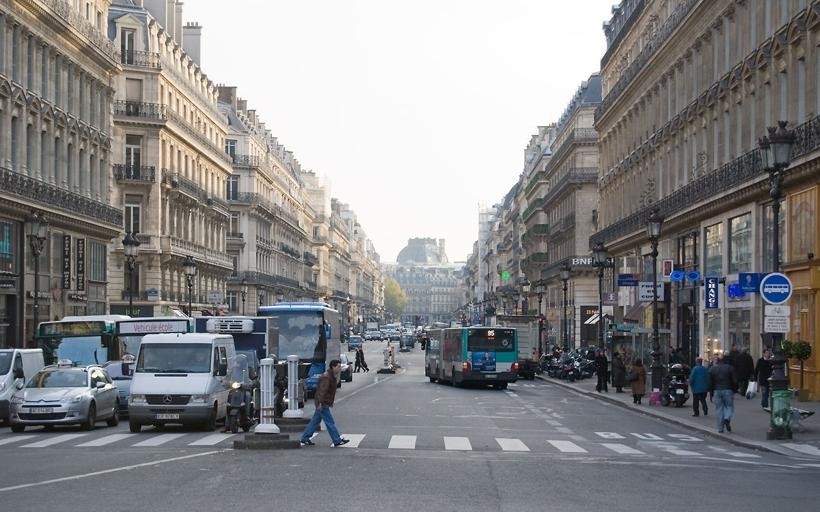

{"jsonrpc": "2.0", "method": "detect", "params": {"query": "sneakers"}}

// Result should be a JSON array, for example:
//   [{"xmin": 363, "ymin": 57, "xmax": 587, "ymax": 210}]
[
  {"xmin": 723, "ymin": 419, "xmax": 732, "ymax": 432},
  {"xmin": 633, "ymin": 397, "xmax": 638, "ymax": 403},
  {"xmin": 637, "ymin": 397, "xmax": 641, "ymax": 405},
  {"xmin": 718, "ymin": 431, "xmax": 724, "ymax": 434}
]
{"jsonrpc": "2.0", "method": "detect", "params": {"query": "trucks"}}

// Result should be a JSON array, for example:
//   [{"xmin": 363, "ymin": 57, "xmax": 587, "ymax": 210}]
[
  {"xmin": 0, "ymin": 347, "xmax": 46, "ymax": 429},
  {"xmin": 121, "ymin": 331, "xmax": 238, "ymax": 435},
  {"xmin": 486, "ymin": 313, "xmax": 545, "ymax": 382}
]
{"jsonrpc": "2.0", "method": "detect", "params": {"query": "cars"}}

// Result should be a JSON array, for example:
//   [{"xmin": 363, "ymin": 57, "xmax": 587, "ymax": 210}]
[
  {"xmin": 339, "ymin": 318, "xmax": 450, "ymax": 384},
  {"xmin": 8, "ymin": 358, "xmax": 122, "ymax": 434},
  {"xmin": 102, "ymin": 358, "xmax": 135, "ymax": 422}
]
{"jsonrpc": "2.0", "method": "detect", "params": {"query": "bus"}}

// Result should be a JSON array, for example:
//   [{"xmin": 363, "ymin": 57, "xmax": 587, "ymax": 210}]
[{"xmin": 422, "ymin": 323, "xmax": 522, "ymax": 390}]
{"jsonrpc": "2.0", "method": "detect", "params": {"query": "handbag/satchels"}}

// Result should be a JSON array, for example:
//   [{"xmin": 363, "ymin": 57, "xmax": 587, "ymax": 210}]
[
  {"xmin": 627, "ymin": 371, "xmax": 640, "ymax": 383},
  {"xmin": 744, "ymin": 380, "xmax": 758, "ymax": 401}
]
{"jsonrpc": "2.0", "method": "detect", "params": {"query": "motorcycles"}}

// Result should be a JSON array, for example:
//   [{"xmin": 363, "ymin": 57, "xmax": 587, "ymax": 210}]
[
  {"xmin": 660, "ymin": 361, "xmax": 690, "ymax": 411},
  {"xmin": 534, "ymin": 344, "xmax": 600, "ymax": 384}
]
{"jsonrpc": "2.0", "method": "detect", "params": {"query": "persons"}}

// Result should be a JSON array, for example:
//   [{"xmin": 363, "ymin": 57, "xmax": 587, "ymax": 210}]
[
  {"xmin": 352, "ymin": 347, "xmax": 366, "ymax": 373},
  {"xmin": 612, "ymin": 352, "xmax": 626, "ymax": 393},
  {"xmin": 709, "ymin": 355, "xmax": 736, "ymax": 434},
  {"xmin": 357, "ymin": 345, "xmax": 369, "ymax": 372},
  {"xmin": 628, "ymin": 358, "xmax": 647, "ymax": 404},
  {"xmin": 706, "ymin": 358, "xmax": 719, "ymax": 402},
  {"xmin": 300, "ymin": 358, "xmax": 350, "ymax": 447},
  {"xmin": 741, "ymin": 348, "xmax": 754, "ymax": 396},
  {"xmin": 754, "ymin": 348, "xmax": 772, "ymax": 411},
  {"xmin": 595, "ymin": 350, "xmax": 609, "ymax": 392},
  {"xmin": 687, "ymin": 356, "xmax": 710, "ymax": 418}
]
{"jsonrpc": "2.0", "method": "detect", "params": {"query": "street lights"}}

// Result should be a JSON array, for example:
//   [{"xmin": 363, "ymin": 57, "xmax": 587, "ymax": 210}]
[
  {"xmin": 644, "ymin": 206, "xmax": 670, "ymax": 395},
  {"xmin": 554, "ymin": 258, "xmax": 574, "ymax": 380},
  {"xmin": 120, "ymin": 228, "xmax": 142, "ymax": 318},
  {"xmin": 757, "ymin": 116, "xmax": 798, "ymax": 441},
  {"xmin": 182, "ymin": 253, "xmax": 197, "ymax": 315},
  {"xmin": 458, "ymin": 277, "xmax": 548, "ymax": 358},
  {"xmin": 240, "ymin": 279, "xmax": 329, "ymax": 315},
  {"xmin": 24, "ymin": 206, "xmax": 52, "ymax": 334},
  {"xmin": 589, "ymin": 239, "xmax": 614, "ymax": 397}
]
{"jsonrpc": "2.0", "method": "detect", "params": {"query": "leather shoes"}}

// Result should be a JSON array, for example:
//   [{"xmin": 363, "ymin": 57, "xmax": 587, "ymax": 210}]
[
  {"xmin": 300, "ymin": 439, "xmax": 315, "ymax": 445},
  {"xmin": 334, "ymin": 439, "xmax": 350, "ymax": 447}
]
